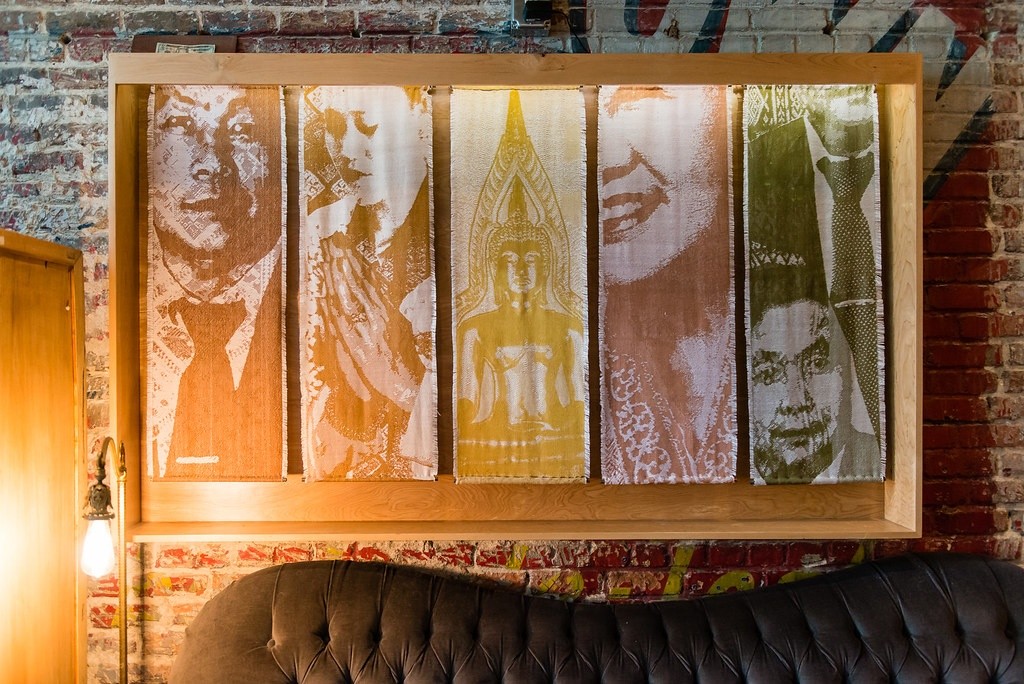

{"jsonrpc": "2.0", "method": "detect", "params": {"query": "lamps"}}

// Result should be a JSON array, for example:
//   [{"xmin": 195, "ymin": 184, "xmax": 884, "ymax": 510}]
[{"xmin": 73, "ymin": 435, "xmax": 129, "ymax": 684}]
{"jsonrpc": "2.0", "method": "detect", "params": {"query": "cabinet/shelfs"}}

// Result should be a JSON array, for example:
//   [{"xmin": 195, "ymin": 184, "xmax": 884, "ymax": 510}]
[{"xmin": 1, "ymin": 230, "xmax": 89, "ymax": 684}]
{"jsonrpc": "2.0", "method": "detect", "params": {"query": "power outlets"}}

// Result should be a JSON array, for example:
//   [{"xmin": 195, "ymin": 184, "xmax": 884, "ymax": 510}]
[{"xmin": 509, "ymin": 0, "xmax": 551, "ymax": 36}]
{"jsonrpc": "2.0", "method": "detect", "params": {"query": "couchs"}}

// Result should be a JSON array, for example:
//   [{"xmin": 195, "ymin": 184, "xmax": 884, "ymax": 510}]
[{"xmin": 172, "ymin": 553, "xmax": 1024, "ymax": 684}]
